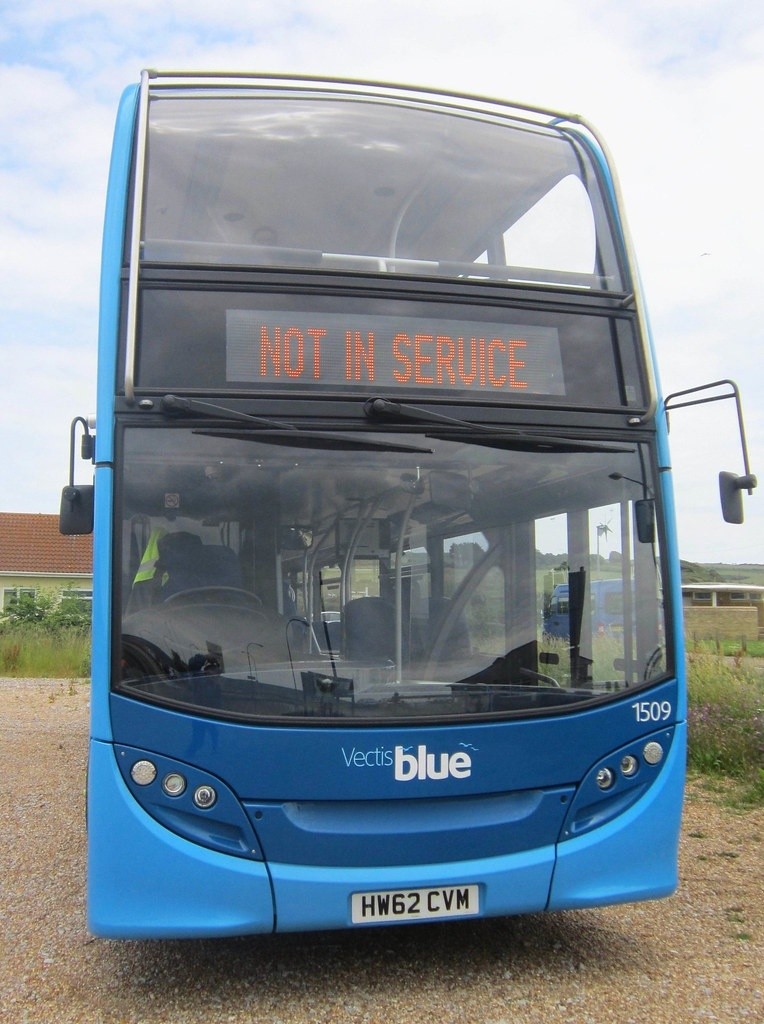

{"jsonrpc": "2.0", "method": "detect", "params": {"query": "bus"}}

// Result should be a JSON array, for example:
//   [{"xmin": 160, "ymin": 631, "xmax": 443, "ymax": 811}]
[{"xmin": 56, "ymin": 66, "xmax": 758, "ymax": 945}]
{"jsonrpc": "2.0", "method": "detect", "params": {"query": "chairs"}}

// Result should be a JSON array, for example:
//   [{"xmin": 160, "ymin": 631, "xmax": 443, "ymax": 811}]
[{"xmin": 146, "ymin": 583, "xmax": 468, "ymax": 662}]
